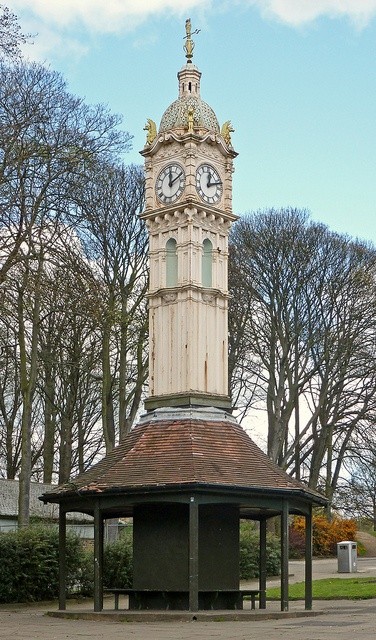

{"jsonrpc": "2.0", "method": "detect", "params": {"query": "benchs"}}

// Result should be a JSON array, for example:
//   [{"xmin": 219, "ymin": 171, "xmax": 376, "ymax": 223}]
[
  {"xmin": 104, "ymin": 589, "xmax": 189, "ymax": 610},
  {"xmin": 198, "ymin": 590, "xmax": 265, "ymax": 610}
]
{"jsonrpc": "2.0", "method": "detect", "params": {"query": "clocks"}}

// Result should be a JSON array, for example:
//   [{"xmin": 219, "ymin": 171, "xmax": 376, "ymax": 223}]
[
  {"xmin": 195, "ymin": 164, "xmax": 224, "ymax": 205},
  {"xmin": 155, "ymin": 163, "xmax": 186, "ymax": 205}
]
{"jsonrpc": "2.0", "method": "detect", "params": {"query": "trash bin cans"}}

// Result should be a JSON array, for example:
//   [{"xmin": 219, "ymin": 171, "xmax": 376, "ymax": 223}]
[{"xmin": 336, "ymin": 541, "xmax": 357, "ymax": 573}]
